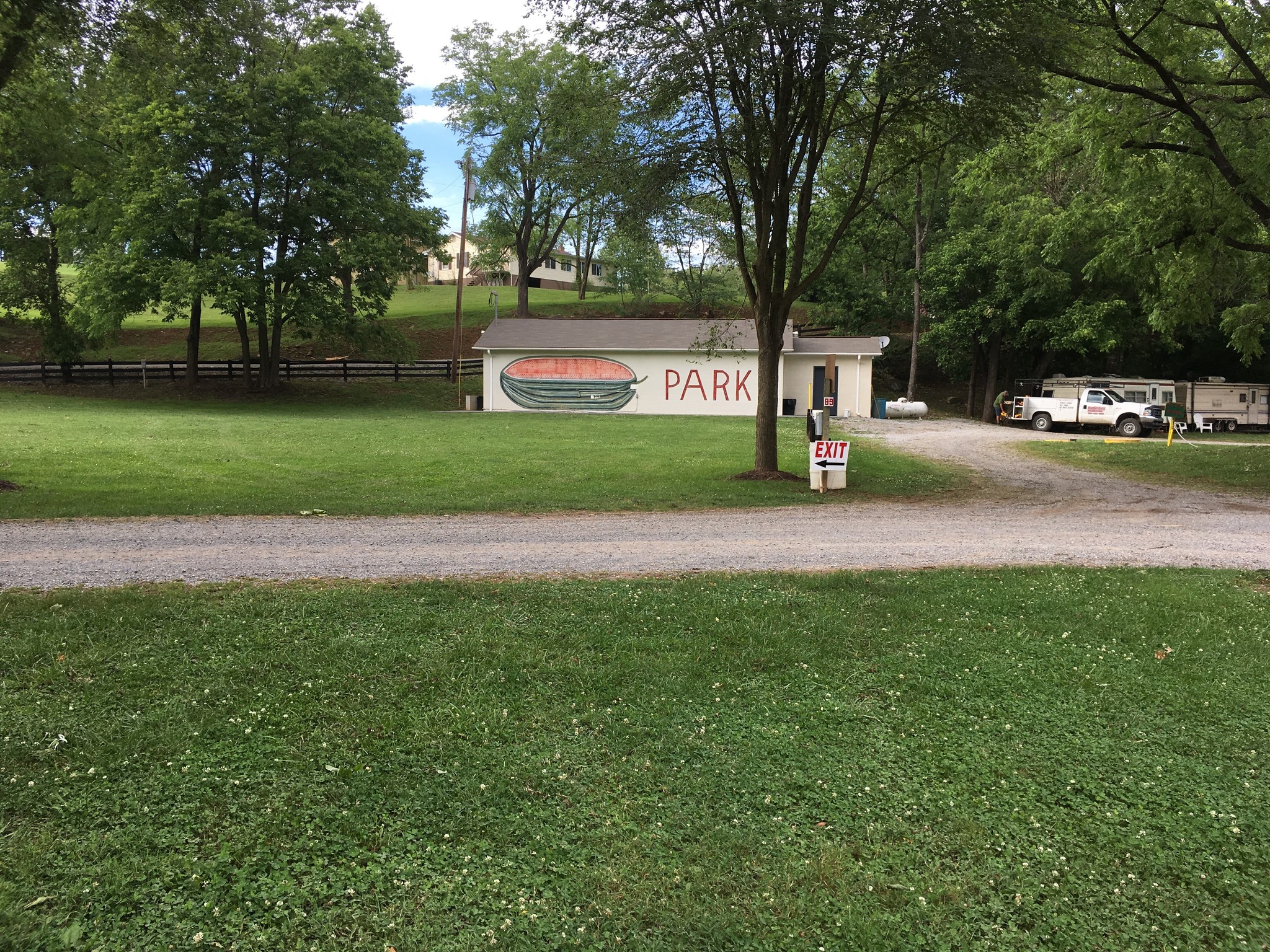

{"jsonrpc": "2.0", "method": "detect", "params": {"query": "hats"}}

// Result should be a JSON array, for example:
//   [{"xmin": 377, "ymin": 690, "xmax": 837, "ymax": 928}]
[{"xmin": 1004, "ymin": 391, "xmax": 1008, "ymax": 394}]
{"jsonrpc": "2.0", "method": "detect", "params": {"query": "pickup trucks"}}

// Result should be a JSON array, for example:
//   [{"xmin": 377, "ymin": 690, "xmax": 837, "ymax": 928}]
[{"xmin": 1006, "ymin": 386, "xmax": 1164, "ymax": 438}]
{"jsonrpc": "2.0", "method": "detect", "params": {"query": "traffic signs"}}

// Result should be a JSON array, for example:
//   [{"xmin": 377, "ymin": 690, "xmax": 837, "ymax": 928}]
[{"xmin": 811, "ymin": 437, "xmax": 851, "ymax": 473}]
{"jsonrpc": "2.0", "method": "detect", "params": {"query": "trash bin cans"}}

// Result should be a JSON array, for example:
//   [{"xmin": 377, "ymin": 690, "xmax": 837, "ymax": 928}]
[
  {"xmin": 477, "ymin": 396, "xmax": 483, "ymax": 410},
  {"xmin": 783, "ymin": 399, "xmax": 796, "ymax": 415},
  {"xmin": 871, "ymin": 398, "xmax": 887, "ymax": 419}
]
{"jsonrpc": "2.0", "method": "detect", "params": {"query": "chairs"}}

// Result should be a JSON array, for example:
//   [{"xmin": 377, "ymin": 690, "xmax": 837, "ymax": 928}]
[
  {"xmin": 1168, "ymin": 416, "xmax": 1187, "ymax": 434},
  {"xmin": 1193, "ymin": 413, "xmax": 1213, "ymax": 433}
]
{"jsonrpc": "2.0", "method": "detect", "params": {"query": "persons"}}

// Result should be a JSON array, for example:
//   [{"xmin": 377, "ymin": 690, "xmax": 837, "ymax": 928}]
[{"xmin": 993, "ymin": 391, "xmax": 1009, "ymax": 426}]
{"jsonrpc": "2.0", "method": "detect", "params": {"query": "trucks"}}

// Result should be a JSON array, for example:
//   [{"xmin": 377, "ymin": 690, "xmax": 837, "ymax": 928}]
[
  {"xmin": 1185, "ymin": 375, "xmax": 1270, "ymax": 434},
  {"xmin": 1040, "ymin": 373, "xmax": 1177, "ymax": 434}
]
